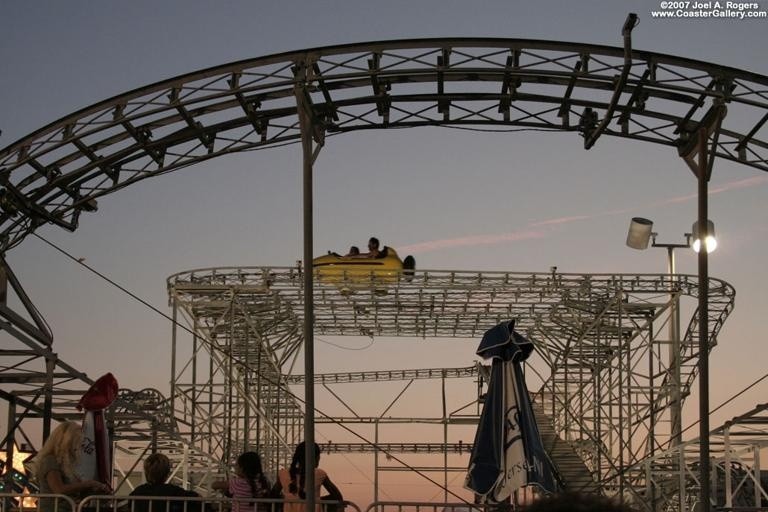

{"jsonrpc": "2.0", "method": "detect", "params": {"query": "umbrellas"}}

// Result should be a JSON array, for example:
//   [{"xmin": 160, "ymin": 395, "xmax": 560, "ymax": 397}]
[
  {"xmin": 461, "ymin": 318, "xmax": 564, "ymax": 512},
  {"xmin": 75, "ymin": 373, "xmax": 119, "ymax": 504}
]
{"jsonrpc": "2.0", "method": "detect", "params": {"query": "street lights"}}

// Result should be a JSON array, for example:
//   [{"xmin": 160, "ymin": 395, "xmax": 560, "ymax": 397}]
[{"xmin": 623, "ymin": 215, "xmax": 719, "ymax": 510}]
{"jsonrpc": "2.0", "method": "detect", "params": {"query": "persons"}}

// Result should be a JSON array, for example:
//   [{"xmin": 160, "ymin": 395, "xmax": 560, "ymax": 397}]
[
  {"xmin": 210, "ymin": 451, "xmax": 272, "ymax": 512},
  {"xmin": 126, "ymin": 452, "xmax": 213, "ymax": 512},
  {"xmin": 24, "ymin": 420, "xmax": 112, "ymax": 512},
  {"xmin": 350, "ymin": 245, "xmax": 359, "ymax": 258},
  {"xmin": 266, "ymin": 440, "xmax": 342, "ymax": 512},
  {"xmin": 349, "ymin": 236, "xmax": 382, "ymax": 259}
]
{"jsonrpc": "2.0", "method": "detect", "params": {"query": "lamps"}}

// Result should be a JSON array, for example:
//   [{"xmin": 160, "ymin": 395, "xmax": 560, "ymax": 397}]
[{"xmin": 626, "ymin": 217, "xmax": 718, "ymax": 254}]
